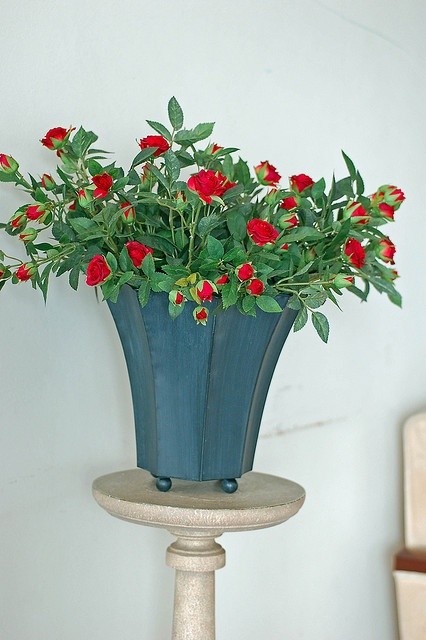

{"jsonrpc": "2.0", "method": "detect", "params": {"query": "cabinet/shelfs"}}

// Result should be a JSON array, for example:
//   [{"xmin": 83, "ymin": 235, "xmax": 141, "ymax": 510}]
[{"xmin": 93, "ymin": 466, "xmax": 304, "ymax": 640}]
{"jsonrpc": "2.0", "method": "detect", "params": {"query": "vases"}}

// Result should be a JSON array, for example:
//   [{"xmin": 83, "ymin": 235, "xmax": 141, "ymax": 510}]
[{"xmin": 98, "ymin": 277, "xmax": 304, "ymax": 492}]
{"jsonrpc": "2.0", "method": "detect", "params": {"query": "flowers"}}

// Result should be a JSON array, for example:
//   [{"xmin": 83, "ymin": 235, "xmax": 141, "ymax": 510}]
[{"xmin": 1, "ymin": 95, "xmax": 406, "ymax": 343}]
